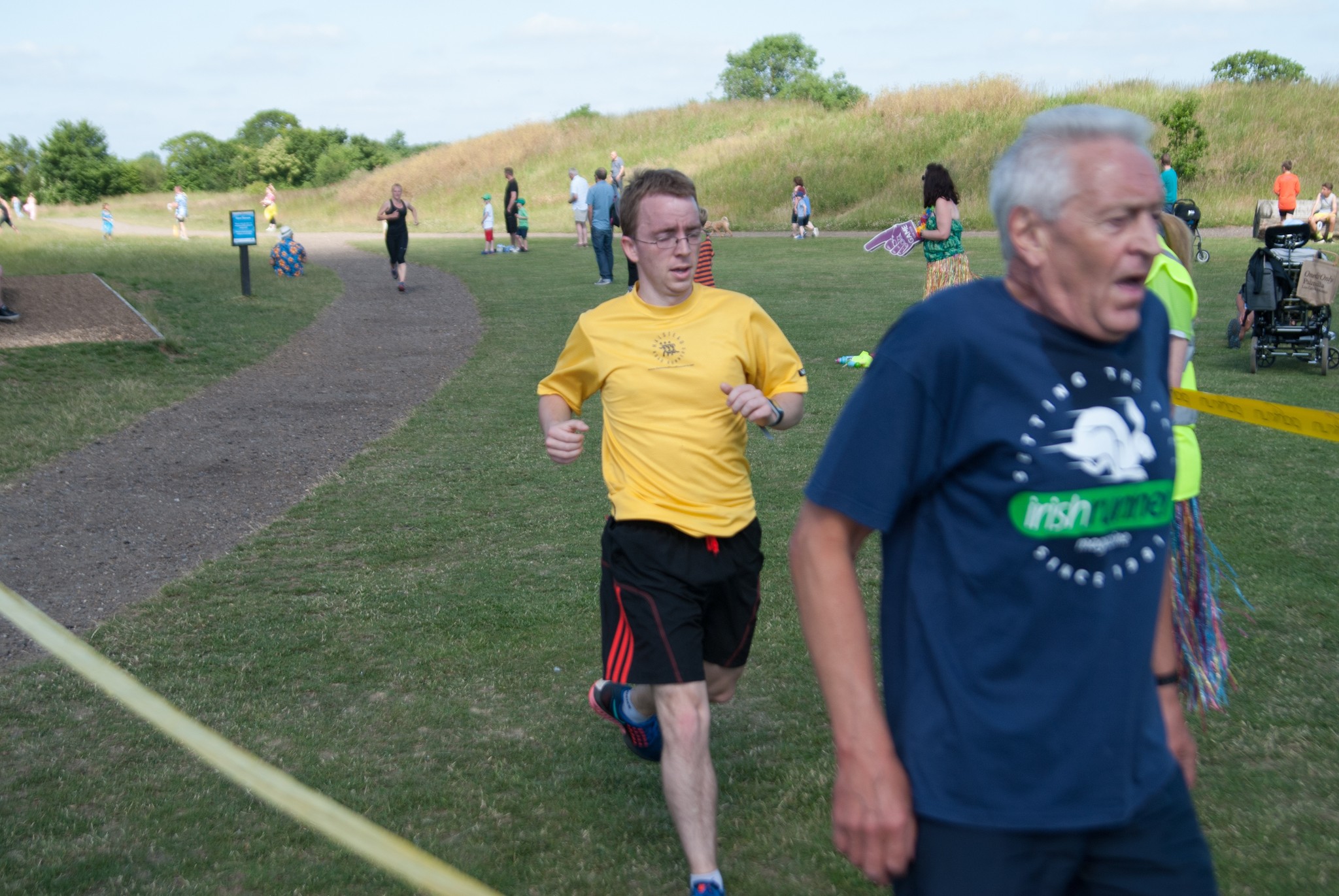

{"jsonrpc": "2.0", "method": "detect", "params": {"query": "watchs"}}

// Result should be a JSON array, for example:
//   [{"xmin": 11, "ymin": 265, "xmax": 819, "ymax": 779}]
[{"xmin": 755, "ymin": 396, "xmax": 785, "ymax": 439}]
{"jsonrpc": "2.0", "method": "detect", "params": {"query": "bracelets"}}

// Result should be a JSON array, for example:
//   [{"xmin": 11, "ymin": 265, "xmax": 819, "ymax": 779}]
[
  {"xmin": 1153, "ymin": 669, "xmax": 1180, "ymax": 687},
  {"xmin": 920, "ymin": 229, "xmax": 925, "ymax": 238}
]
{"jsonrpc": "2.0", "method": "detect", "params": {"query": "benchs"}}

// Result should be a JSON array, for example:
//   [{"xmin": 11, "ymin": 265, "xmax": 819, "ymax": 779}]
[{"xmin": 1253, "ymin": 200, "xmax": 1339, "ymax": 239}]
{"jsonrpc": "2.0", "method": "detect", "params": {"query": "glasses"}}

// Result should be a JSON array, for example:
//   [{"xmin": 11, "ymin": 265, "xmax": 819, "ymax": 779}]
[
  {"xmin": 922, "ymin": 174, "xmax": 926, "ymax": 180},
  {"xmin": 631, "ymin": 231, "xmax": 709, "ymax": 248}
]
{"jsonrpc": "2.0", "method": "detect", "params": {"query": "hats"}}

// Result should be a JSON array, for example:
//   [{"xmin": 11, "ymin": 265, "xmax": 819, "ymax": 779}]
[
  {"xmin": 481, "ymin": 194, "xmax": 492, "ymax": 199},
  {"xmin": 281, "ymin": 226, "xmax": 293, "ymax": 238},
  {"xmin": 514, "ymin": 198, "xmax": 525, "ymax": 204},
  {"xmin": 795, "ymin": 191, "xmax": 804, "ymax": 198},
  {"xmin": 798, "ymin": 186, "xmax": 805, "ymax": 194}
]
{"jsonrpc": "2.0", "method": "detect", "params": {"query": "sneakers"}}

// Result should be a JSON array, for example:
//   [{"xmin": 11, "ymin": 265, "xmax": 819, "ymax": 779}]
[
  {"xmin": 594, "ymin": 278, "xmax": 613, "ymax": 285},
  {"xmin": 1322, "ymin": 326, "xmax": 1335, "ymax": 340},
  {"xmin": 398, "ymin": 282, "xmax": 405, "ymax": 291},
  {"xmin": 589, "ymin": 678, "xmax": 663, "ymax": 762},
  {"xmin": 391, "ymin": 263, "xmax": 398, "ymax": 279},
  {"xmin": 1227, "ymin": 318, "xmax": 1240, "ymax": 349},
  {"xmin": 690, "ymin": 881, "xmax": 725, "ymax": 896}
]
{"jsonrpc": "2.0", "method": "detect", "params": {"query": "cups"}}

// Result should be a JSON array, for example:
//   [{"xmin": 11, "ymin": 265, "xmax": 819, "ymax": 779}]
[{"xmin": 167, "ymin": 203, "xmax": 171, "ymax": 210}]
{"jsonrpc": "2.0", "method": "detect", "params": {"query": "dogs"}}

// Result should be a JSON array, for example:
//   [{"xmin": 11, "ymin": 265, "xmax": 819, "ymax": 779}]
[{"xmin": 703, "ymin": 216, "xmax": 733, "ymax": 237}]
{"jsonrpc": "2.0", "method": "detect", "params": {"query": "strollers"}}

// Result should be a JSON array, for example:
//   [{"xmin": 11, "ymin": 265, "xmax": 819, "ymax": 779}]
[
  {"xmin": 1240, "ymin": 222, "xmax": 1339, "ymax": 378},
  {"xmin": 1165, "ymin": 198, "xmax": 1210, "ymax": 264}
]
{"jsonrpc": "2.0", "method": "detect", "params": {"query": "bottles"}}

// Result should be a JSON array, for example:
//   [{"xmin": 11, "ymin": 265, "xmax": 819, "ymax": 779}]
[
  {"xmin": 497, "ymin": 244, "xmax": 504, "ymax": 252},
  {"xmin": 836, "ymin": 355, "xmax": 854, "ymax": 364}
]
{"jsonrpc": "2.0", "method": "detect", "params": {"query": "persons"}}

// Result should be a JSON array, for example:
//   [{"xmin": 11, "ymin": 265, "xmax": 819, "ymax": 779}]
[
  {"xmin": 610, "ymin": 152, "xmax": 626, "ymax": 196},
  {"xmin": 625, "ymin": 257, "xmax": 639, "ymax": 294},
  {"xmin": 1161, "ymin": 153, "xmax": 1177, "ymax": 217},
  {"xmin": 1273, "ymin": 160, "xmax": 1299, "ymax": 225},
  {"xmin": 270, "ymin": 226, "xmax": 308, "ymax": 277},
  {"xmin": 1308, "ymin": 182, "xmax": 1338, "ymax": 245},
  {"xmin": 694, "ymin": 207, "xmax": 716, "ymax": 289},
  {"xmin": 529, "ymin": 166, "xmax": 816, "ymax": 896},
  {"xmin": 168, "ymin": 186, "xmax": 189, "ymax": 240},
  {"xmin": 0, "ymin": 193, "xmax": 37, "ymax": 234},
  {"xmin": 375, "ymin": 183, "xmax": 419, "ymax": 292},
  {"xmin": 794, "ymin": 112, "xmax": 1222, "ymax": 896},
  {"xmin": 586, "ymin": 167, "xmax": 619, "ymax": 286},
  {"xmin": 794, "ymin": 187, "xmax": 819, "ymax": 238},
  {"xmin": 789, "ymin": 176, "xmax": 814, "ymax": 237},
  {"xmin": 503, "ymin": 167, "xmax": 519, "ymax": 246},
  {"xmin": 568, "ymin": 167, "xmax": 589, "ymax": 248},
  {"xmin": 1226, "ymin": 224, "xmax": 1328, "ymax": 350},
  {"xmin": 480, "ymin": 195, "xmax": 495, "ymax": 254},
  {"xmin": 914, "ymin": 161, "xmax": 973, "ymax": 302},
  {"xmin": 261, "ymin": 186, "xmax": 277, "ymax": 232},
  {"xmin": 102, "ymin": 203, "xmax": 114, "ymax": 240},
  {"xmin": 514, "ymin": 197, "xmax": 529, "ymax": 251},
  {"xmin": 795, "ymin": 191, "xmax": 815, "ymax": 238},
  {"xmin": 1140, "ymin": 238, "xmax": 1239, "ymax": 709}
]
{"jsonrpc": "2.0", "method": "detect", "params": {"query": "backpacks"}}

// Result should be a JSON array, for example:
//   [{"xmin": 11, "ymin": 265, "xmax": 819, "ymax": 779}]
[{"xmin": 1241, "ymin": 260, "xmax": 1282, "ymax": 310}]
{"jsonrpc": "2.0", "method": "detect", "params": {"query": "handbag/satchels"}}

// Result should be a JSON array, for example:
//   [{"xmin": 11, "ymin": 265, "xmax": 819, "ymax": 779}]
[
  {"xmin": 610, "ymin": 185, "xmax": 620, "ymax": 227},
  {"xmin": 1295, "ymin": 249, "xmax": 1339, "ymax": 307}
]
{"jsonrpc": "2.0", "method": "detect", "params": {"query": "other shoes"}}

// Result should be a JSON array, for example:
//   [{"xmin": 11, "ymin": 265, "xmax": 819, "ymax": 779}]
[
  {"xmin": 790, "ymin": 227, "xmax": 818, "ymax": 238},
  {"xmin": 572, "ymin": 243, "xmax": 583, "ymax": 247},
  {"xmin": 0, "ymin": 305, "xmax": 20, "ymax": 319},
  {"xmin": 1326, "ymin": 238, "xmax": 1334, "ymax": 243},
  {"xmin": 584, "ymin": 243, "xmax": 588, "ymax": 247},
  {"xmin": 1314, "ymin": 238, "xmax": 1325, "ymax": 243},
  {"xmin": 266, "ymin": 227, "xmax": 275, "ymax": 231},
  {"xmin": 481, "ymin": 246, "xmax": 528, "ymax": 254}
]
